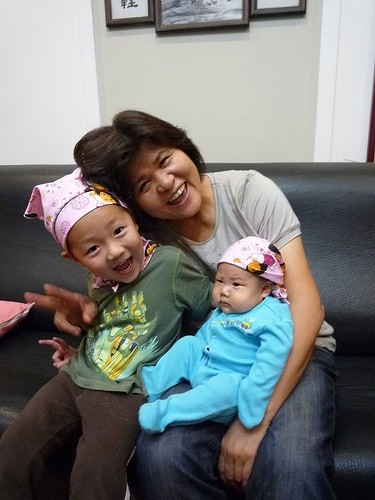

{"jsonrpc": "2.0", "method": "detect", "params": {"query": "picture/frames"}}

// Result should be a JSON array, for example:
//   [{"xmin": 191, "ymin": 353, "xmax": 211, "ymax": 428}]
[
  {"xmin": 250, "ymin": 0, "xmax": 306, "ymax": 18},
  {"xmin": 105, "ymin": 0, "xmax": 154, "ymax": 28},
  {"xmin": 154, "ymin": 0, "xmax": 250, "ymax": 34}
]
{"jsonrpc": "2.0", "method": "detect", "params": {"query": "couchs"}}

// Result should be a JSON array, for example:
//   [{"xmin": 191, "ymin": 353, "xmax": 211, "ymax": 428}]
[{"xmin": 0, "ymin": 163, "xmax": 375, "ymax": 500}]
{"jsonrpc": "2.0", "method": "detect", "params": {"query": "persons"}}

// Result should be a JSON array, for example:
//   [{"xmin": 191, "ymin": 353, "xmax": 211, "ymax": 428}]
[
  {"xmin": 138, "ymin": 236, "xmax": 293, "ymax": 434},
  {"xmin": 24, "ymin": 109, "xmax": 338, "ymax": 500},
  {"xmin": 0, "ymin": 167, "xmax": 217, "ymax": 500}
]
